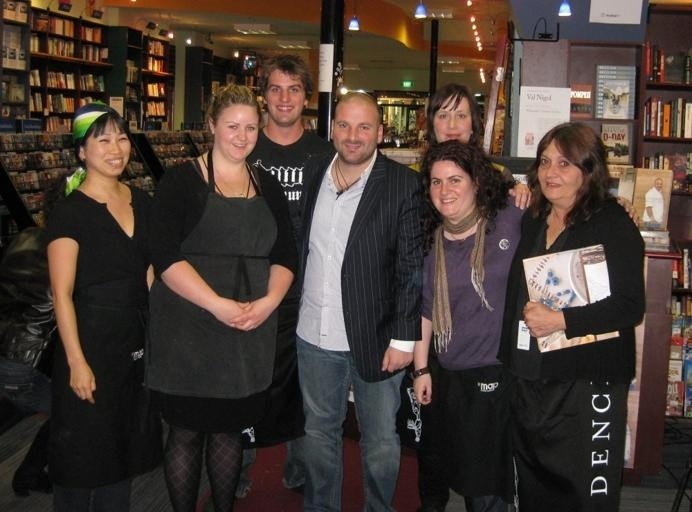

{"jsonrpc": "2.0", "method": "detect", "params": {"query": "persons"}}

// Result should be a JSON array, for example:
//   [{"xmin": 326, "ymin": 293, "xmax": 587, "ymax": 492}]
[
  {"xmin": 12, "ymin": 104, "xmax": 154, "ymax": 512},
  {"xmin": 498, "ymin": 123, "xmax": 644, "ymax": 512},
  {"xmin": 297, "ymin": 92, "xmax": 424, "ymax": 512},
  {"xmin": 147, "ymin": 85, "xmax": 294, "ymax": 512},
  {"xmin": 642, "ymin": 178, "xmax": 664, "ymax": 230},
  {"xmin": 0, "ymin": 176, "xmax": 82, "ymax": 498},
  {"xmin": 232, "ymin": 54, "xmax": 341, "ymax": 501},
  {"xmin": 409, "ymin": 82, "xmax": 533, "ymax": 211},
  {"xmin": 413, "ymin": 140, "xmax": 528, "ymax": 512}
]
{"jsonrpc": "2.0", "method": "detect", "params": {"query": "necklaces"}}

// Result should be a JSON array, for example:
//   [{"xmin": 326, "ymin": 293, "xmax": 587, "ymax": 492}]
[
  {"xmin": 214, "ymin": 162, "xmax": 246, "ymax": 198},
  {"xmin": 333, "ymin": 157, "xmax": 362, "ymax": 199}
]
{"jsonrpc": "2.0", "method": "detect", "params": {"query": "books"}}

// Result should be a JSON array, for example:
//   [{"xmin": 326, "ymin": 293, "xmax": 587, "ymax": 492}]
[
  {"xmin": 522, "ymin": 244, "xmax": 620, "ymax": 353},
  {"xmin": 29, "ymin": 11, "xmax": 108, "ymax": 133},
  {"xmin": 0, "ymin": 0, "xmax": 27, "ymax": 131},
  {"xmin": 517, "ymin": 86, "xmax": 571, "ymax": 157},
  {"xmin": 607, "ymin": 163, "xmax": 634, "ymax": 209},
  {"xmin": 595, "ymin": 66, "xmax": 635, "ymax": 120},
  {"xmin": 645, "ymin": 152, "xmax": 692, "ymax": 194},
  {"xmin": 639, "ymin": 230, "xmax": 671, "ymax": 251},
  {"xmin": 125, "ymin": 61, "xmax": 145, "ymax": 130},
  {"xmin": 632, "ymin": 168, "xmax": 673, "ymax": 233},
  {"xmin": 644, "ymin": 42, "xmax": 691, "ymax": 85},
  {"xmin": 645, "ymin": 97, "xmax": 692, "ymax": 139},
  {"xmin": 569, "ymin": 84, "xmax": 594, "ymax": 121},
  {"xmin": 146, "ymin": 40, "xmax": 170, "ymax": 130},
  {"xmin": 665, "ymin": 249, "xmax": 692, "ymax": 418}
]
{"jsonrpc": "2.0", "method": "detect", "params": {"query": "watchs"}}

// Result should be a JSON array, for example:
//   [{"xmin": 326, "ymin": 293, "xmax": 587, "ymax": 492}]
[{"xmin": 413, "ymin": 367, "xmax": 430, "ymax": 379}]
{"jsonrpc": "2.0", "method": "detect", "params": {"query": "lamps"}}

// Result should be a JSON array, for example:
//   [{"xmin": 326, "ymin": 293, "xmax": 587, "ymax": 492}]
[
  {"xmin": 414, "ymin": 0, "xmax": 428, "ymax": 19},
  {"xmin": 348, "ymin": 0, "xmax": 361, "ymax": 31},
  {"xmin": 559, "ymin": 0, "xmax": 573, "ymax": 17}
]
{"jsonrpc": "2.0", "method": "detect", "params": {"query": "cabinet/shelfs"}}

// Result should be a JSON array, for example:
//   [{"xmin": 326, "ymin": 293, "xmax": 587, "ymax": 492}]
[
  {"xmin": 185, "ymin": 125, "xmax": 214, "ymax": 155},
  {"xmin": 138, "ymin": 128, "xmax": 197, "ymax": 176},
  {"xmin": 632, "ymin": 3, "xmax": 692, "ymax": 427},
  {"xmin": 0, "ymin": 126, "xmax": 155, "ymax": 234},
  {"xmin": 1, "ymin": 0, "xmax": 174, "ymax": 133},
  {"xmin": 183, "ymin": 46, "xmax": 261, "ymax": 131}
]
